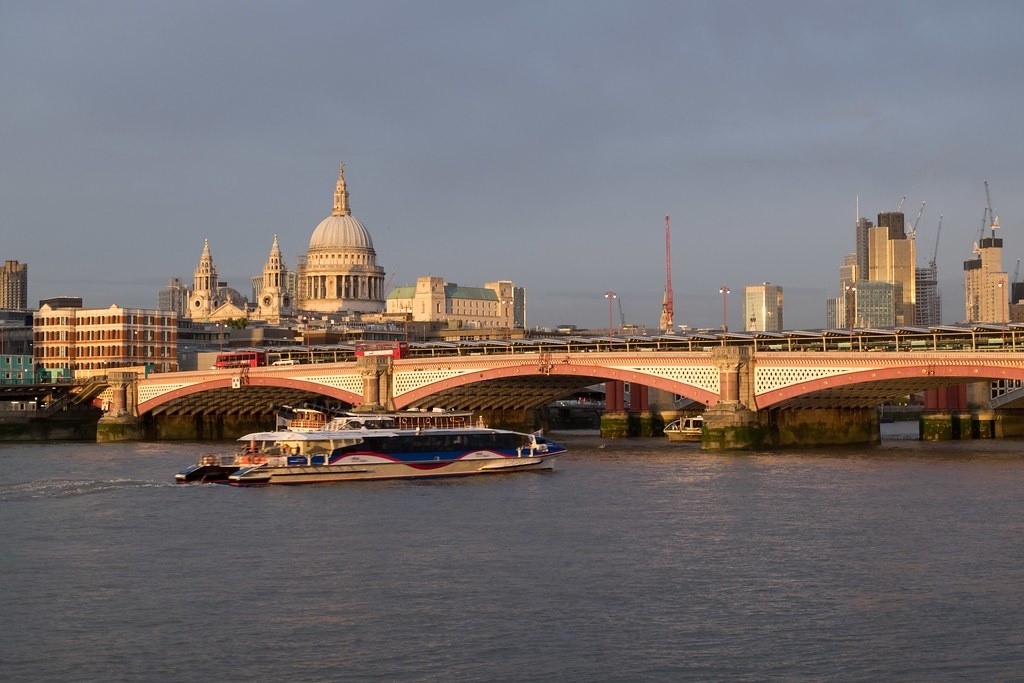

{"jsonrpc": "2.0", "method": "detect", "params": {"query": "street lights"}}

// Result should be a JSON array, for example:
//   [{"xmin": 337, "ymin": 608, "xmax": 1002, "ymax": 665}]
[
  {"xmin": 605, "ymin": 288, "xmax": 617, "ymax": 352},
  {"xmin": 501, "ymin": 297, "xmax": 513, "ymax": 354},
  {"xmin": 998, "ymin": 277, "xmax": 1006, "ymax": 352},
  {"xmin": 401, "ymin": 304, "xmax": 412, "ymax": 358},
  {"xmin": 303, "ymin": 316, "xmax": 315, "ymax": 364},
  {"xmin": 719, "ymin": 283, "xmax": 730, "ymax": 347},
  {"xmin": 846, "ymin": 281, "xmax": 856, "ymax": 351}
]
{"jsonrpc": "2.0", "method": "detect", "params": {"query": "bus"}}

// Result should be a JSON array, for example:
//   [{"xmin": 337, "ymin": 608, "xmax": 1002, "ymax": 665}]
[
  {"xmin": 354, "ymin": 341, "xmax": 409, "ymax": 361},
  {"xmin": 213, "ymin": 351, "xmax": 267, "ymax": 370}
]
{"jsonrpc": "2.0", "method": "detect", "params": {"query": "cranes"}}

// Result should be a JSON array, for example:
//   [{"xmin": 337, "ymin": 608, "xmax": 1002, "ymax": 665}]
[
  {"xmin": 663, "ymin": 214, "xmax": 678, "ymax": 334},
  {"xmin": 855, "ymin": 178, "xmax": 1021, "ymax": 307}
]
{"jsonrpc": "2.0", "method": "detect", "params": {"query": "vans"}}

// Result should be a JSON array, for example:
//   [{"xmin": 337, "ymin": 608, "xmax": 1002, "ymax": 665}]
[{"xmin": 272, "ymin": 360, "xmax": 300, "ymax": 366}]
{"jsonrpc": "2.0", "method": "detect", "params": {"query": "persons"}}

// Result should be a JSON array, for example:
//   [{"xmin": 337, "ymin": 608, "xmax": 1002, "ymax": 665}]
[{"xmin": 281, "ymin": 442, "xmax": 300, "ymax": 457}]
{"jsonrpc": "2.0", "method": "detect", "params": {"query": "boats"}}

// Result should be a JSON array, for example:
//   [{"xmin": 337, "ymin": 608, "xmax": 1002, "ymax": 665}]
[
  {"xmin": 663, "ymin": 415, "xmax": 704, "ymax": 442},
  {"xmin": 173, "ymin": 401, "xmax": 570, "ymax": 488}
]
